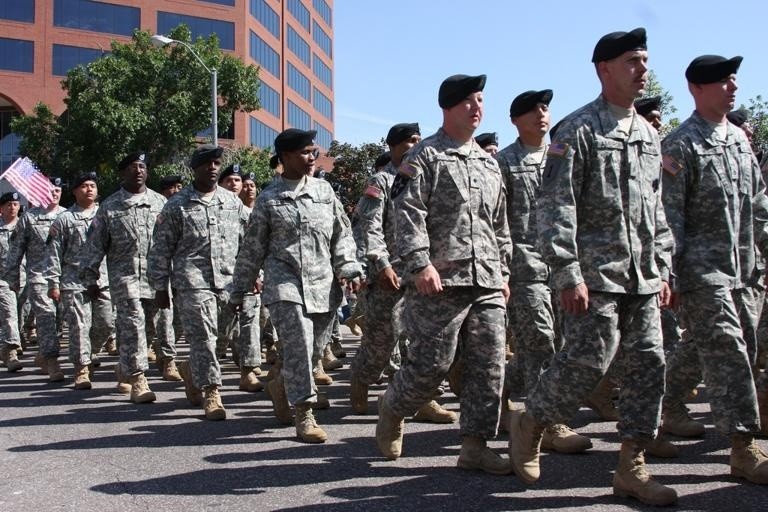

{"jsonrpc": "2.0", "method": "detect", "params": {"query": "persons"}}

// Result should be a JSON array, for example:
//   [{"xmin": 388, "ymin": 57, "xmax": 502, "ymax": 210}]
[
  {"xmin": 0, "ymin": 174, "xmax": 115, "ymax": 390},
  {"xmin": 348, "ymin": 25, "xmax": 768, "ymax": 508},
  {"xmin": 79, "ymin": 127, "xmax": 368, "ymax": 446}
]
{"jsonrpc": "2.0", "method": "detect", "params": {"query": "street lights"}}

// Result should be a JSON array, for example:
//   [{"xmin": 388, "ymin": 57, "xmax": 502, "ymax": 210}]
[{"xmin": 151, "ymin": 33, "xmax": 218, "ymax": 148}]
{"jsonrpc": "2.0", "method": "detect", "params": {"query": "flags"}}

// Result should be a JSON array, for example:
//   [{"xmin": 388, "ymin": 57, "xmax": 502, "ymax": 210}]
[{"xmin": 3, "ymin": 157, "xmax": 55, "ymax": 209}]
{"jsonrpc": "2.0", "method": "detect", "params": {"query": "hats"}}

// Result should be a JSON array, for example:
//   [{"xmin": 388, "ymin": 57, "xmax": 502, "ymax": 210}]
[
  {"xmin": 159, "ymin": 176, "xmax": 186, "ymax": 192},
  {"xmin": 509, "ymin": 90, "xmax": 552, "ymax": 117},
  {"xmin": 438, "ymin": 74, "xmax": 487, "ymax": 109},
  {"xmin": 0, "ymin": 192, "xmax": 22, "ymax": 205},
  {"xmin": 635, "ymin": 95, "xmax": 661, "ymax": 113},
  {"xmin": 686, "ymin": 56, "xmax": 743, "ymax": 84},
  {"xmin": 273, "ymin": 128, "xmax": 317, "ymax": 151},
  {"xmin": 475, "ymin": 132, "xmax": 498, "ymax": 149},
  {"xmin": 373, "ymin": 124, "xmax": 420, "ymax": 168},
  {"xmin": 47, "ymin": 177, "xmax": 63, "ymax": 187},
  {"xmin": 591, "ymin": 28, "xmax": 647, "ymax": 62},
  {"xmin": 191, "ymin": 144, "xmax": 256, "ymax": 180},
  {"xmin": 118, "ymin": 151, "xmax": 153, "ymax": 171},
  {"xmin": 71, "ymin": 171, "xmax": 97, "ymax": 188}
]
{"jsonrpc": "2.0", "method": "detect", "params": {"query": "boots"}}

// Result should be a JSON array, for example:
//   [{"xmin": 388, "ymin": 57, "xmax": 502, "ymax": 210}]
[
  {"xmin": 541, "ymin": 422, "xmax": 593, "ymax": 451},
  {"xmin": 351, "ymin": 372, "xmax": 456, "ymax": 457},
  {"xmin": 2, "ymin": 326, "xmax": 38, "ymax": 370},
  {"xmin": 581, "ymin": 377, "xmax": 620, "ymax": 421},
  {"xmin": 612, "ymin": 440, "xmax": 679, "ymax": 506},
  {"xmin": 457, "ymin": 435, "xmax": 511, "ymax": 474},
  {"xmin": 238, "ymin": 316, "xmax": 360, "ymax": 443},
  {"xmin": 35, "ymin": 354, "xmax": 101, "ymax": 390},
  {"xmin": 661, "ymin": 403, "xmax": 706, "ymax": 437},
  {"xmin": 161, "ymin": 357, "xmax": 227, "ymax": 421},
  {"xmin": 509, "ymin": 413, "xmax": 546, "ymax": 484},
  {"xmin": 731, "ymin": 431, "xmax": 768, "ymax": 487},
  {"xmin": 105, "ymin": 336, "xmax": 156, "ymax": 404},
  {"xmin": 754, "ymin": 390, "xmax": 768, "ymax": 440},
  {"xmin": 499, "ymin": 388, "xmax": 517, "ymax": 429}
]
